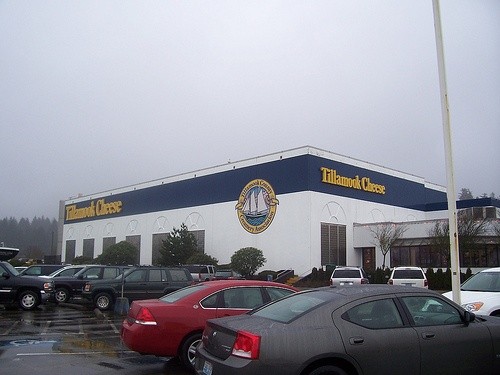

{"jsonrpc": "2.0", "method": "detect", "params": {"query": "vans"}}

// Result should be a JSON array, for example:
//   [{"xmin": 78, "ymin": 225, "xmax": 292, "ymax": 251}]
[{"xmin": 181, "ymin": 263, "xmax": 216, "ymax": 283}]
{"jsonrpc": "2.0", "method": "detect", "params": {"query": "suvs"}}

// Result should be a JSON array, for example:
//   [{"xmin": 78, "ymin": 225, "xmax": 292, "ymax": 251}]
[
  {"xmin": 0, "ymin": 260, "xmax": 55, "ymax": 312},
  {"xmin": 81, "ymin": 267, "xmax": 200, "ymax": 312},
  {"xmin": 386, "ymin": 266, "xmax": 429, "ymax": 290},
  {"xmin": 329, "ymin": 265, "xmax": 369, "ymax": 286}
]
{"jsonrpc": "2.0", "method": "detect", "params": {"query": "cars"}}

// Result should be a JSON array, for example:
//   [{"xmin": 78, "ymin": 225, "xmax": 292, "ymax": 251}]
[
  {"xmin": 422, "ymin": 267, "xmax": 500, "ymax": 319},
  {"xmin": 194, "ymin": 283, "xmax": 500, "ymax": 375},
  {"xmin": 120, "ymin": 280, "xmax": 326, "ymax": 372},
  {"xmin": 14, "ymin": 264, "xmax": 134, "ymax": 304}
]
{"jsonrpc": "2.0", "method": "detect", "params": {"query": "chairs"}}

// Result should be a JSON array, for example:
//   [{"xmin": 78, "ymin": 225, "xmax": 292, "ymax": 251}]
[
  {"xmin": 229, "ymin": 295, "xmax": 240, "ymax": 308},
  {"xmin": 374, "ymin": 302, "xmax": 397, "ymax": 327},
  {"xmin": 245, "ymin": 295, "xmax": 258, "ymax": 308}
]
